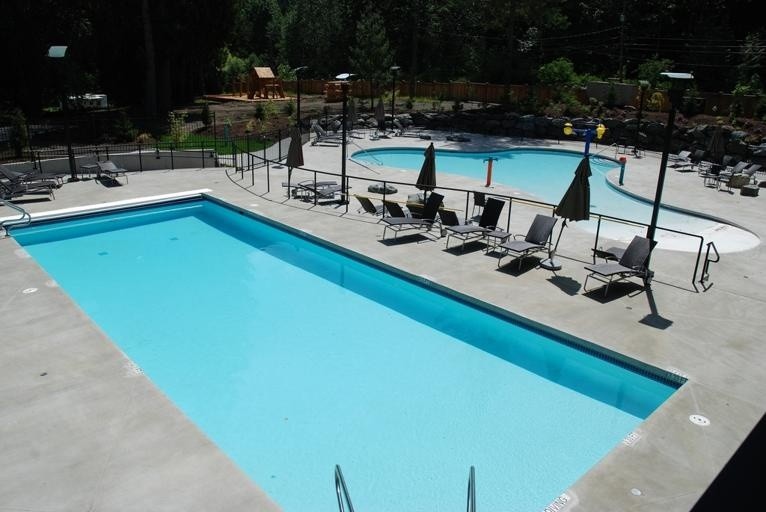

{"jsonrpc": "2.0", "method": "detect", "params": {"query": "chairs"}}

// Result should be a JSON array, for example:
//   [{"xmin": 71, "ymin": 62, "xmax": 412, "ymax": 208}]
[
  {"xmin": 0, "ymin": 160, "xmax": 128, "ymax": 201},
  {"xmin": 497, "ymin": 213, "xmax": 558, "ymax": 274},
  {"xmin": 581, "ymin": 234, "xmax": 658, "ymax": 297},
  {"xmin": 282, "ymin": 181, "xmax": 353, "ymax": 207},
  {"xmin": 356, "ymin": 191, "xmax": 512, "ymax": 256},
  {"xmin": 617, "ymin": 136, "xmax": 645, "ymax": 158},
  {"xmin": 311, "ymin": 120, "xmax": 425, "ymax": 146},
  {"xmin": 670, "ymin": 149, "xmax": 762, "ymax": 197}
]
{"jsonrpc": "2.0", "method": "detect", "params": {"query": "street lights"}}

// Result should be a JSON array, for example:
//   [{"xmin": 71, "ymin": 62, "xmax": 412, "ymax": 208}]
[
  {"xmin": 640, "ymin": 69, "xmax": 696, "ymax": 273},
  {"xmin": 288, "ymin": 66, "xmax": 308, "ymax": 131},
  {"xmin": 390, "ymin": 66, "xmax": 400, "ymax": 131},
  {"xmin": 44, "ymin": 44, "xmax": 79, "ymax": 182},
  {"xmin": 336, "ymin": 73, "xmax": 359, "ymax": 205},
  {"xmin": 619, "ymin": 12, "xmax": 626, "ymax": 84}
]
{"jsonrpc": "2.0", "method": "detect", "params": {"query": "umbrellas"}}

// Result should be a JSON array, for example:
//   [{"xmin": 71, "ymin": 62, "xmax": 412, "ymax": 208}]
[
  {"xmin": 413, "ymin": 141, "xmax": 437, "ymax": 205},
  {"xmin": 707, "ymin": 122, "xmax": 727, "ymax": 161},
  {"xmin": 373, "ymin": 95, "xmax": 386, "ymax": 122},
  {"xmin": 536, "ymin": 156, "xmax": 594, "ymax": 270},
  {"xmin": 284, "ymin": 124, "xmax": 304, "ymax": 168}
]
